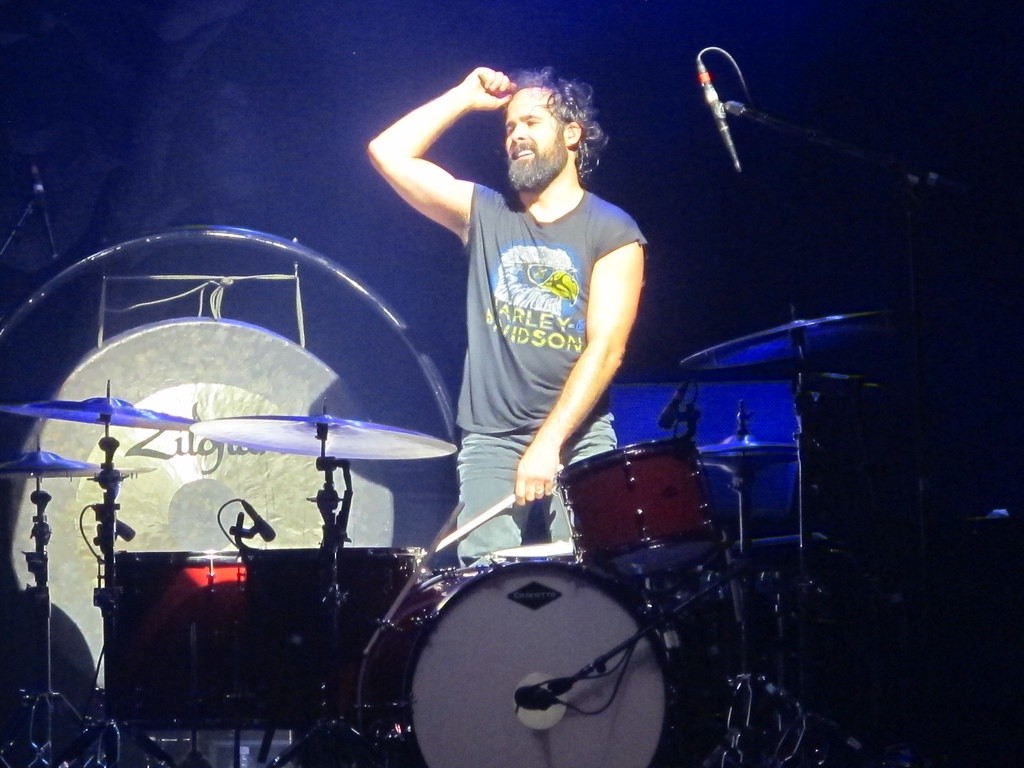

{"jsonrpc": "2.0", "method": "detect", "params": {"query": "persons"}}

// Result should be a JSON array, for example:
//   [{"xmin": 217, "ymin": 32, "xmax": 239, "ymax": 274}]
[{"xmin": 365, "ymin": 51, "xmax": 649, "ymax": 569}]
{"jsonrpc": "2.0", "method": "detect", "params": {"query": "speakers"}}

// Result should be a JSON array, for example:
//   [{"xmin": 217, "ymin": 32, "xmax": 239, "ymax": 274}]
[{"xmin": 609, "ymin": 380, "xmax": 801, "ymax": 526}]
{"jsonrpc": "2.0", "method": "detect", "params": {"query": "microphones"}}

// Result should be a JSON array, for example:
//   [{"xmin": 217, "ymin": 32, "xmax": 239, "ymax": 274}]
[
  {"xmin": 697, "ymin": 59, "xmax": 744, "ymax": 172},
  {"xmin": 516, "ymin": 685, "xmax": 553, "ymax": 711},
  {"xmin": 242, "ymin": 499, "xmax": 276, "ymax": 542},
  {"xmin": 659, "ymin": 382, "xmax": 689, "ymax": 429},
  {"xmin": 92, "ymin": 504, "xmax": 136, "ymax": 541}
]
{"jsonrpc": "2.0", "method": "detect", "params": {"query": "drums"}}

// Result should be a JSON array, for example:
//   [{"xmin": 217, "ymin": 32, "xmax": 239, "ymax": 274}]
[
  {"xmin": 103, "ymin": 542, "xmax": 425, "ymax": 728},
  {"xmin": 354, "ymin": 540, "xmax": 671, "ymax": 768},
  {"xmin": 556, "ymin": 434, "xmax": 724, "ymax": 577},
  {"xmin": 489, "ymin": 540, "xmax": 575, "ymax": 567}
]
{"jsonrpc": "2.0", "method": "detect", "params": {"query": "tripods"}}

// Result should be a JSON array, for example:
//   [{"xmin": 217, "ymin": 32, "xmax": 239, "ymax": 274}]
[
  {"xmin": 0, "ymin": 423, "xmax": 176, "ymax": 768},
  {"xmin": 270, "ymin": 454, "xmax": 383, "ymax": 768},
  {"xmin": 703, "ymin": 455, "xmax": 888, "ymax": 768}
]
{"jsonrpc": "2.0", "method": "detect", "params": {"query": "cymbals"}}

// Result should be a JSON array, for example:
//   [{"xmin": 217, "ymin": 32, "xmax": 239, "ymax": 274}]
[
  {"xmin": 189, "ymin": 412, "xmax": 458, "ymax": 462},
  {"xmin": 690, "ymin": 438, "xmax": 800, "ymax": 471},
  {"xmin": 1, "ymin": 450, "xmax": 156, "ymax": 481},
  {"xmin": 0, "ymin": 396, "xmax": 192, "ymax": 432},
  {"xmin": 679, "ymin": 309, "xmax": 888, "ymax": 374}
]
